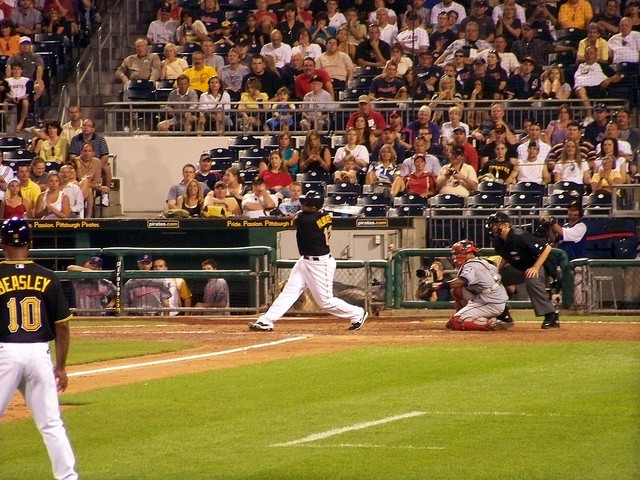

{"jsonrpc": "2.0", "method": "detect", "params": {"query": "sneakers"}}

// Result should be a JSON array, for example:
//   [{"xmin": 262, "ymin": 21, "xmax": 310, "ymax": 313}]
[
  {"xmin": 348, "ymin": 311, "xmax": 368, "ymax": 330},
  {"xmin": 102, "ymin": 193, "xmax": 109, "ymax": 206},
  {"xmin": 579, "ymin": 117, "xmax": 594, "ymax": 127},
  {"xmin": 489, "ymin": 318, "xmax": 509, "ymax": 330},
  {"xmin": 248, "ymin": 322, "xmax": 273, "ymax": 331},
  {"xmin": 541, "ymin": 313, "xmax": 560, "ymax": 329},
  {"xmin": 497, "ymin": 306, "xmax": 513, "ymax": 323}
]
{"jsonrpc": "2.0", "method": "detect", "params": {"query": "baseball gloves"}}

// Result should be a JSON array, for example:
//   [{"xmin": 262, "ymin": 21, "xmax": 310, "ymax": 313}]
[{"xmin": 416, "ymin": 281, "xmax": 433, "ymax": 299}]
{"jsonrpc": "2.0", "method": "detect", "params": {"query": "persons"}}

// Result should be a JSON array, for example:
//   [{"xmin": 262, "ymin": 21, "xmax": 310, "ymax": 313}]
[
  {"xmin": 511, "ymin": 140, "xmax": 551, "ymax": 186},
  {"xmin": 201, "ymin": 179, "xmax": 242, "ymax": 218},
  {"xmin": 4, "ymin": 60, "xmax": 33, "ymax": 132},
  {"xmin": 213, "ymin": 19, "xmax": 238, "ymax": 53},
  {"xmin": 401, "ymin": 0, "xmax": 430, "ymax": 27},
  {"xmin": 314, "ymin": 36, "xmax": 355, "ymax": 93},
  {"xmin": 577, "ymin": 22, "xmax": 609, "ymax": 63},
  {"xmin": 241, "ymin": 177, "xmax": 277, "ymax": 218},
  {"xmin": 74, "ymin": 0, "xmax": 91, "ymax": 24},
  {"xmin": 294, "ymin": 57, "xmax": 335, "ymax": 102},
  {"xmin": 430, "ymin": 9, "xmax": 460, "ymax": 33},
  {"xmin": 254, "ymin": 0, "xmax": 278, "ymax": 34},
  {"xmin": 282, "ymin": 0, "xmax": 312, "ymax": 30},
  {"xmin": 122, "ymin": 255, "xmax": 170, "ymax": 316},
  {"xmin": 247, "ymin": 190, "xmax": 368, "ymax": 332},
  {"xmin": 4, "ymin": 164, "xmax": 42, "ymax": 218},
  {"xmin": 298, "ymin": 74, "xmax": 334, "ymax": 133},
  {"xmin": 434, "ymin": 144, "xmax": 478, "ymax": 197},
  {"xmin": 405, "ymin": 67, "xmax": 430, "ymax": 101},
  {"xmin": 474, "ymin": 138, "xmax": 516, "ymax": 186},
  {"xmin": 236, "ymin": 75, "xmax": 268, "ymax": 132},
  {"xmin": 355, "ymin": 23, "xmax": 390, "ymax": 68},
  {"xmin": 430, "ymin": 0, "xmax": 466, "ymax": 26},
  {"xmin": 282, "ymin": 52, "xmax": 304, "ymax": 101},
  {"xmin": 528, "ymin": 66, "xmax": 571, "ymax": 100},
  {"xmin": 176, "ymin": 7, "xmax": 209, "ymax": 45},
  {"xmin": 66, "ymin": 255, "xmax": 116, "ymax": 315},
  {"xmin": 336, "ymin": 28, "xmax": 356, "ymax": 62},
  {"xmin": 428, "ymin": 72, "xmax": 464, "ymax": 123},
  {"xmin": 510, "ymin": 20, "xmax": 577, "ymax": 64},
  {"xmin": 458, "ymin": 0, "xmax": 495, "ymax": 43},
  {"xmin": 308, "ymin": 11, "xmax": 336, "ymax": 52},
  {"xmin": 506, "ymin": 55, "xmax": 541, "ymax": 100},
  {"xmin": 73, "ymin": 142, "xmax": 111, "ymax": 218},
  {"xmin": 314, "ymin": 0, "xmax": 346, "ymax": 33},
  {"xmin": 165, "ymin": 163, "xmax": 209, "ymax": 209},
  {"xmin": 623, "ymin": 2, "xmax": 640, "ymax": 32},
  {"xmin": 156, "ymin": 0, "xmax": 183, "ymax": 24},
  {"xmin": 485, "ymin": 210, "xmax": 559, "ymax": 329},
  {"xmin": 198, "ymin": 37, "xmax": 224, "ymax": 70},
  {"xmin": 332, "ymin": 153, "xmax": 360, "ymax": 186},
  {"xmin": 412, "ymin": 126, "xmax": 442, "ymax": 156},
  {"xmin": 196, "ymin": 152, "xmax": 220, "ymax": 189},
  {"xmin": 29, "ymin": 156, "xmax": 49, "ymax": 191},
  {"xmin": 260, "ymin": 28, "xmax": 291, "ymax": 67},
  {"xmin": 545, "ymin": 121, "xmax": 596, "ymax": 170},
  {"xmin": 441, "ymin": 106, "xmax": 470, "ymax": 147},
  {"xmin": 278, "ymin": 182, "xmax": 305, "ymax": 217},
  {"xmin": 241, "ymin": 54, "xmax": 274, "ymax": 96},
  {"xmin": 414, "ymin": 49, "xmax": 441, "ymax": 91},
  {"xmin": 345, "ymin": 93, "xmax": 385, "ymax": 144},
  {"xmin": 276, "ymin": 3, "xmax": 305, "ymax": 47},
  {"xmin": 68, "ymin": 118, "xmax": 112, "ymax": 207},
  {"xmin": 471, "ymin": 56, "xmax": 485, "ymax": 78},
  {"xmin": 257, "ymin": 130, "xmax": 298, "ymax": 175},
  {"xmin": 217, "ymin": 47, "xmax": 250, "ymax": 93},
  {"xmin": 559, "ymin": 0, "xmax": 594, "ymax": 30},
  {"xmin": 345, "ymin": 0, "xmax": 370, "ymax": 23},
  {"xmin": 589, "ymin": 153, "xmax": 622, "ymax": 198},
  {"xmin": 172, "ymin": 50, "xmax": 219, "ymax": 97},
  {"xmin": 175, "ymin": 178, "xmax": 204, "ymax": 219},
  {"xmin": 444, "ymin": 125, "xmax": 478, "ymax": 172},
  {"xmin": 368, "ymin": 60, "xmax": 407, "ymax": 102},
  {"xmin": 291, "ymin": 31, "xmax": 322, "ymax": 62},
  {"xmin": 39, "ymin": 120, "xmax": 68, "ymax": 165},
  {"xmin": 590, "ymin": 0, "xmax": 621, "ymax": 40},
  {"xmin": 429, "ymin": 10, "xmax": 457, "ymax": 62},
  {"xmin": 264, "ymin": 86, "xmax": 295, "ymax": 131},
  {"xmin": 194, "ymin": 0, "xmax": 225, "ymax": 39},
  {"xmin": 0, "ymin": 178, "xmax": 31, "ymax": 219},
  {"xmin": 0, "ymin": 215, "xmax": 81, "ymax": 478},
  {"xmin": 453, "ymin": 49, "xmax": 471, "ymax": 85},
  {"xmin": 240, "ymin": 10, "xmax": 265, "ymax": 53},
  {"xmin": 400, "ymin": 151, "xmax": 436, "ymax": 199},
  {"xmin": 159, "ymin": 42, "xmax": 189, "ymax": 79},
  {"xmin": 443, "ymin": 62, "xmax": 464, "ymax": 95},
  {"xmin": 42, "ymin": 4, "xmax": 72, "ymax": 38},
  {"xmin": 334, "ymin": 126, "xmax": 370, "ymax": 170},
  {"xmin": 114, "ymin": 38, "xmax": 162, "ymax": 85},
  {"xmin": 374, "ymin": 8, "xmax": 398, "ymax": 47},
  {"xmin": 146, "ymin": 2, "xmax": 179, "ymax": 52},
  {"xmin": 570, "ymin": 44, "xmax": 621, "ymax": 126},
  {"xmin": 152, "ymin": 256, "xmax": 192, "ymax": 315},
  {"xmin": 192, "ymin": 258, "xmax": 230, "ymax": 316},
  {"xmin": 0, "ymin": 18, "xmax": 20, "ymax": 56},
  {"xmin": 582, "ymin": 103, "xmax": 608, "ymax": 145},
  {"xmin": 430, "ymin": 239, "xmax": 513, "ymax": 331},
  {"xmin": 157, "ymin": 74, "xmax": 199, "ymax": 132},
  {"xmin": 480, "ymin": 123, "xmax": 517, "ymax": 160},
  {"xmin": 61, "ymin": 104, "xmax": 83, "ymax": 144},
  {"xmin": 484, "ymin": 50, "xmax": 507, "ymax": 100},
  {"xmin": 544, "ymin": 103, "xmax": 571, "ymax": 144},
  {"xmin": 407, "ymin": 105, "xmax": 440, "ymax": 146},
  {"xmin": 224, "ymin": 166, "xmax": 244, "ymax": 198},
  {"xmin": 516, "ymin": 118, "xmax": 546, "ymax": 143},
  {"xmin": 495, "ymin": 1, "xmax": 522, "ymax": 52},
  {"xmin": 526, "ymin": 0, "xmax": 558, "ymax": 27},
  {"xmin": 348, "ymin": 114, "xmax": 376, "ymax": 151},
  {"xmin": 235, "ymin": 37, "xmax": 252, "ymax": 69},
  {"xmin": 385, "ymin": 0, "xmax": 401, "ymax": 15},
  {"xmin": 592, "ymin": 136, "xmax": 626, "ymax": 183},
  {"xmin": 307, "ymin": 0, "xmax": 327, "ymax": 18},
  {"xmin": 255, "ymin": 151, "xmax": 294, "ymax": 189},
  {"xmin": 59, "ymin": 164, "xmax": 83, "ymax": 217},
  {"xmin": 370, "ymin": 123, "xmax": 406, "ymax": 163},
  {"xmin": 606, "ymin": 17, "xmax": 640, "ymax": 101},
  {"xmin": 517, "ymin": 121, "xmax": 551, "ymax": 161},
  {"xmin": 552, "ymin": 137, "xmax": 591, "ymax": 184},
  {"xmin": 541, "ymin": 200, "xmax": 588, "ymax": 293},
  {"xmin": 596, "ymin": 119, "xmax": 634, "ymax": 161},
  {"xmin": 65, "ymin": 161, "xmax": 94, "ymax": 218},
  {"xmin": 339, "ymin": 8, "xmax": 366, "ymax": 59},
  {"xmin": 0, "ymin": 148, "xmax": 14, "ymax": 202},
  {"xmin": 394, "ymin": 10, "xmax": 430, "ymax": 66},
  {"xmin": 196, "ymin": 75, "xmax": 231, "ymax": 136},
  {"xmin": 34, "ymin": 169, "xmax": 69, "ymax": 218},
  {"xmin": 7, "ymin": 0, "xmax": 42, "ymax": 34},
  {"xmin": 469, "ymin": 78, "xmax": 486, "ymax": 108},
  {"xmin": 5, "ymin": 35, "xmax": 45, "ymax": 104},
  {"xmin": 491, "ymin": 0, "xmax": 526, "ymax": 27},
  {"xmin": 365, "ymin": 143, "xmax": 405, "ymax": 197},
  {"xmin": 470, "ymin": 34, "xmax": 521, "ymax": 75},
  {"xmin": 27, "ymin": 124, "xmax": 48, "ymax": 151},
  {"xmin": 383, "ymin": 42, "xmax": 413, "ymax": 74},
  {"xmin": 433, "ymin": 21, "xmax": 493, "ymax": 66},
  {"xmin": 382, "ymin": 109, "xmax": 415, "ymax": 149},
  {"xmin": 470, "ymin": 101, "xmax": 517, "ymax": 143},
  {"xmin": 367, "ymin": 0, "xmax": 397, "ymax": 25},
  {"xmin": 607, "ymin": 108, "xmax": 640, "ymax": 151},
  {"xmin": 298, "ymin": 129, "xmax": 332, "ymax": 172},
  {"xmin": 399, "ymin": 135, "xmax": 443, "ymax": 180}
]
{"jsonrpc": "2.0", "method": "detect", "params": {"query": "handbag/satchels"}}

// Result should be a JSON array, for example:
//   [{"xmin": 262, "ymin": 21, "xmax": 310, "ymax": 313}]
[
  {"xmin": 201, "ymin": 205, "xmax": 231, "ymax": 220},
  {"xmin": 159, "ymin": 209, "xmax": 190, "ymax": 218}
]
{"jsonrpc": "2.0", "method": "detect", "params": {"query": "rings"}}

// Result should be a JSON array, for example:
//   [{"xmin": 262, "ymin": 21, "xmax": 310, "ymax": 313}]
[{"xmin": 104, "ymin": 190, "xmax": 109, "ymax": 195}]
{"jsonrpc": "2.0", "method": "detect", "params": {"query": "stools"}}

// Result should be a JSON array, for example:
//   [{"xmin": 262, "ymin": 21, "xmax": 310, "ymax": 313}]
[{"xmin": 593, "ymin": 274, "xmax": 617, "ymax": 310}]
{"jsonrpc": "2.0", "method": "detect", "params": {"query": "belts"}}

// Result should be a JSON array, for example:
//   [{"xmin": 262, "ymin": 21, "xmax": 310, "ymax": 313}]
[
  {"xmin": 128, "ymin": 313, "xmax": 162, "ymax": 316},
  {"xmin": 304, "ymin": 253, "xmax": 332, "ymax": 261}
]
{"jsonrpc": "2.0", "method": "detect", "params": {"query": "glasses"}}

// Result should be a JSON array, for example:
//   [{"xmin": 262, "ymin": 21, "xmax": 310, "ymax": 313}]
[
  {"xmin": 581, "ymin": 65, "xmax": 591, "ymax": 74},
  {"xmin": 328, "ymin": 42, "xmax": 337, "ymax": 46},
  {"xmin": 560, "ymin": 111, "xmax": 568, "ymax": 113},
  {"xmin": 420, "ymin": 133, "xmax": 430, "ymax": 135},
  {"xmin": 300, "ymin": 34, "xmax": 307, "ymax": 37},
  {"xmin": 51, "ymin": 147, "xmax": 55, "ymax": 155},
  {"xmin": 391, "ymin": 50, "xmax": 400, "ymax": 54},
  {"xmin": 82, "ymin": 124, "xmax": 93, "ymax": 127},
  {"xmin": 347, "ymin": 133, "xmax": 357, "ymax": 136},
  {"xmin": 442, "ymin": 74, "xmax": 452, "ymax": 77},
  {"xmin": 570, "ymin": 162, "xmax": 575, "ymax": 170},
  {"xmin": 247, "ymin": 77, "xmax": 256, "ymax": 82},
  {"xmin": 1, "ymin": 26, "xmax": 9, "ymax": 28},
  {"xmin": 415, "ymin": 136, "xmax": 425, "ymax": 140},
  {"xmin": 368, "ymin": 30, "xmax": 386, "ymax": 35},
  {"xmin": 439, "ymin": 11, "xmax": 448, "ymax": 16},
  {"xmin": 183, "ymin": 171, "xmax": 194, "ymax": 173},
  {"xmin": 455, "ymin": 54, "xmax": 463, "ymax": 58}
]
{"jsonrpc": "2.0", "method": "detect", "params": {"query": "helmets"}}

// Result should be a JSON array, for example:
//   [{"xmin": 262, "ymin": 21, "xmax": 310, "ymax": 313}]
[
  {"xmin": 452, "ymin": 239, "xmax": 479, "ymax": 253},
  {"xmin": 299, "ymin": 190, "xmax": 324, "ymax": 209},
  {"xmin": 487, "ymin": 211, "xmax": 510, "ymax": 242},
  {"xmin": 0, "ymin": 217, "xmax": 30, "ymax": 247}
]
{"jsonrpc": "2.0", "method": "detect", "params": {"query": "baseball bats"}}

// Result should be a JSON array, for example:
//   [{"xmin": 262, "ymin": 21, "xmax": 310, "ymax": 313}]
[{"xmin": 321, "ymin": 205, "xmax": 364, "ymax": 215}]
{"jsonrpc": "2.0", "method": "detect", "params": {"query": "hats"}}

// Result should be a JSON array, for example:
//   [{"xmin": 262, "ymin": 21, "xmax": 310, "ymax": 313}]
[
  {"xmin": 384, "ymin": 125, "xmax": 394, "ymax": 131},
  {"xmin": 19, "ymin": 36, "xmax": 31, "ymax": 43},
  {"xmin": 473, "ymin": 58, "xmax": 485, "ymax": 66},
  {"xmin": 238, "ymin": 37, "xmax": 248, "ymax": 45},
  {"xmin": 406, "ymin": 11, "xmax": 418, "ymax": 21},
  {"xmin": 452, "ymin": 146, "xmax": 464, "ymax": 154},
  {"xmin": 308, "ymin": 74, "xmax": 323, "ymax": 83},
  {"xmin": 358, "ymin": 95, "xmax": 370, "ymax": 103},
  {"xmin": 528, "ymin": 142, "xmax": 539, "ymax": 149},
  {"xmin": 495, "ymin": 124, "xmax": 505, "ymax": 133},
  {"xmin": 453, "ymin": 127, "xmax": 465, "ymax": 132},
  {"xmin": 521, "ymin": 23, "xmax": 531, "ymax": 30},
  {"xmin": 474, "ymin": 1, "xmax": 486, "ymax": 6},
  {"xmin": 137, "ymin": 255, "xmax": 152, "ymax": 263},
  {"xmin": 213, "ymin": 181, "xmax": 227, "ymax": 188},
  {"xmin": 592, "ymin": 103, "xmax": 607, "ymax": 112},
  {"xmin": 504, "ymin": 0, "xmax": 515, "ymax": 9},
  {"xmin": 252, "ymin": 177, "xmax": 264, "ymax": 185},
  {"xmin": 389, "ymin": 111, "xmax": 401, "ymax": 118},
  {"xmin": 89, "ymin": 257, "xmax": 102, "ymax": 265},
  {"xmin": 566, "ymin": 200, "xmax": 581, "ymax": 208},
  {"xmin": 200, "ymin": 153, "xmax": 212, "ymax": 162},
  {"xmin": 420, "ymin": 52, "xmax": 435, "ymax": 60},
  {"xmin": 48, "ymin": 5, "xmax": 61, "ymax": 12},
  {"xmin": 162, "ymin": 2, "xmax": 171, "ymax": 12},
  {"xmin": 221, "ymin": 20, "xmax": 231, "ymax": 27},
  {"xmin": 522, "ymin": 55, "xmax": 537, "ymax": 66},
  {"xmin": 414, "ymin": 152, "xmax": 425, "ymax": 162}
]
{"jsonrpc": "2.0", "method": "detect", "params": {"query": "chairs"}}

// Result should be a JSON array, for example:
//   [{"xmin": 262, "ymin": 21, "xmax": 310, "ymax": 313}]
[{"xmin": 1, "ymin": 1, "xmax": 101, "ymax": 172}]
